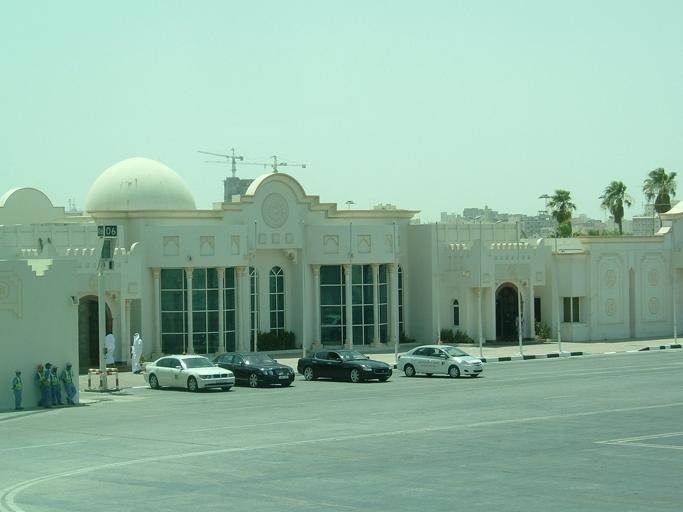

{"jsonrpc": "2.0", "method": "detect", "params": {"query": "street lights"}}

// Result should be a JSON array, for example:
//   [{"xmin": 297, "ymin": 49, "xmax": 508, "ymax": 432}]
[{"xmin": 92, "ymin": 234, "xmax": 120, "ymax": 386}]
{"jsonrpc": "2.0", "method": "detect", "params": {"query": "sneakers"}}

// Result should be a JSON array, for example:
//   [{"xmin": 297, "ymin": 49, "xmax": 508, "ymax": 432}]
[{"xmin": 15, "ymin": 397, "xmax": 76, "ymax": 410}]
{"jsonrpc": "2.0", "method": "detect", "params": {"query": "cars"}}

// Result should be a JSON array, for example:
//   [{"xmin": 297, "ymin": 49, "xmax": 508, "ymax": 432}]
[
  {"xmin": 139, "ymin": 354, "xmax": 238, "ymax": 393},
  {"xmin": 295, "ymin": 348, "xmax": 393, "ymax": 384},
  {"xmin": 210, "ymin": 350, "xmax": 296, "ymax": 390},
  {"xmin": 395, "ymin": 344, "xmax": 484, "ymax": 378}
]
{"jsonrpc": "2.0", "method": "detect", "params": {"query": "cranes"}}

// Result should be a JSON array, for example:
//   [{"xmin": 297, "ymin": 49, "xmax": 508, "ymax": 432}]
[
  {"xmin": 195, "ymin": 144, "xmax": 244, "ymax": 178},
  {"xmin": 201, "ymin": 152, "xmax": 309, "ymax": 174}
]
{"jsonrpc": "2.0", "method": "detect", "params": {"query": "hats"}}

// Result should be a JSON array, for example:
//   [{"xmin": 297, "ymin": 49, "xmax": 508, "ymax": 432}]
[{"xmin": 14, "ymin": 361, "xmax": 73, "ymax": 374}]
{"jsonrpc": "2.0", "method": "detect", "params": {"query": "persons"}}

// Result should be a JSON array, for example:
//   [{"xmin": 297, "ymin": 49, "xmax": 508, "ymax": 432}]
[
  {"xmin": 104, "ymin": 333, "xmax": 115, "ymax": 367},
  {"xmin": 12, "ymin": 371, "xmax": 24, "ymax": 410},
  {"xmin": 35, "ymin": 361, "xmax": 77, "ymax": 408},
  {"xmin": 131, "ymin": 333, "xmax": 142, "ymax": 373}
]
